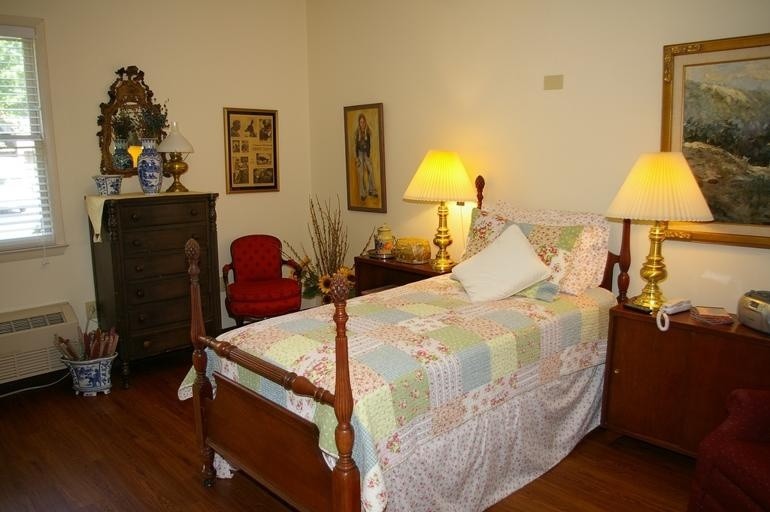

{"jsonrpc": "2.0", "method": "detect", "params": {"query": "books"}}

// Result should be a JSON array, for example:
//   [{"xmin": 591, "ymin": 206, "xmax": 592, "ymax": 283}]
[{"xmin": 689, "ymin": 303, "xmax": 736, "ymax": 326}]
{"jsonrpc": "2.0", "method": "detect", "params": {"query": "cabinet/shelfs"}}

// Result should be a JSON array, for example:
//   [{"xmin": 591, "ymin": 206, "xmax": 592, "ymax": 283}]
[{"xmin": 83, "ymin": 190, "xmax": 223, "ymax": 389}]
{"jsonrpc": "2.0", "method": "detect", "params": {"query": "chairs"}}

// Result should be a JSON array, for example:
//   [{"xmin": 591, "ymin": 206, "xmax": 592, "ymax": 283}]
[
  {"xmin": 222, "ymin": 234, "xmax": 302, "ymax": 329},
  {"xmin": 684, "ymin": 389, "xmax": 770, "ymax": 512}
]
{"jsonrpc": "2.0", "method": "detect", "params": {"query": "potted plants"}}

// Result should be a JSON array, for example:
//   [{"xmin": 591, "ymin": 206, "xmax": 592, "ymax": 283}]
[
  {"xmin": 134, "ymin": 96, "xmax": 169, "ymax": 197},
  {"xmin": 111, "ymin": 102, "xmax": 135, "ymax": 169}
]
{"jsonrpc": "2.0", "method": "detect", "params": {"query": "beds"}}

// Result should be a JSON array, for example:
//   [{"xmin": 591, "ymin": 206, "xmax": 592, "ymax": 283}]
[{"xmin": 183, "ymin": 175, "xmax": 632, "ymax": 512}]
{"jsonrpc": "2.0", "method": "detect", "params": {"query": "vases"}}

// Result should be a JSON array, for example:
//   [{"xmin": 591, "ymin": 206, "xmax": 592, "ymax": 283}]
[
  {"xmin": 60, "ymin": 352, "xmax": 118, "ymax": 398},
  {"xmin": 92, "ymin": 175, "xmax": 123, "ymax": 197}
]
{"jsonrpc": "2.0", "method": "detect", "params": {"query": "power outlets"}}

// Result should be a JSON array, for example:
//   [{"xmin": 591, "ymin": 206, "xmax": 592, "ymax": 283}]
[{"xmin": 84, "ymin": 301, "xmax": 97, "ymax": 321}]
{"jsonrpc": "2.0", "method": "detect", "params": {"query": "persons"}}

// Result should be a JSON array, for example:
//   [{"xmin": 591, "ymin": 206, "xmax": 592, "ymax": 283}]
[{"xmin": 353, "ymin": 113, "xmax": 379, "ymax": 201}]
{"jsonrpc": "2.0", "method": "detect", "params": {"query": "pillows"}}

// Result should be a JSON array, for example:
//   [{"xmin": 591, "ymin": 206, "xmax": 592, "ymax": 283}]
[
  {"xmin": 452, "ymin": 227, "xmax": 552, "ymax": 303},
  {"xmin": 558, "ymin": 224, "xmax": 604, "ymax": 297},
  {"xmin": 491, "ymin": 200, "xmax": 610, "ymax": 289},
  {"xmin": 458, "ymin": 207, "xmax": 583, "ymax": 302}
]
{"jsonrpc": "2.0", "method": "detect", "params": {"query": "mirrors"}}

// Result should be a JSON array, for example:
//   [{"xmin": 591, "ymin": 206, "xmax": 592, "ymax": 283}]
[{"xmin": 96, "ymin": 66, "xmax": 169, "ymax": 179}]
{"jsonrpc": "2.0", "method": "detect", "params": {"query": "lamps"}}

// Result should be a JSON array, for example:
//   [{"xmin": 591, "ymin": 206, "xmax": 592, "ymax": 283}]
[
  {"xmin": 604, "ymin": 150, "xmax": 714, "ymax": 315},
  {"xmin": 403, "ymin": 149, "xmax": 476, "ymax": 275},
  {"xmin": 158, "ymin": 121, "xmax": 194, "ymax": 192}
]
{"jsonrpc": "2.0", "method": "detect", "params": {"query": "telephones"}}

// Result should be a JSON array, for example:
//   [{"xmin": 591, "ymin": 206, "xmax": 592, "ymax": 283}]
[{"xmin": 662, "ymin": 296, "xmax": 692, "ymax": 315}]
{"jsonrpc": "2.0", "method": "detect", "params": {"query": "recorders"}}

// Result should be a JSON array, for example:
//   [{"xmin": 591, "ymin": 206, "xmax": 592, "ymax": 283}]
[{"xmin": 736, "ymin": 289, "xmax": 770, "ymax": 334}]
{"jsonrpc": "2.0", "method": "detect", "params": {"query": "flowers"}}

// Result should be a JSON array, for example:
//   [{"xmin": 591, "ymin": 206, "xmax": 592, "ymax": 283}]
[{"xmin": 278, "ymin": 192, "xmax": 376, "ymax": 308}]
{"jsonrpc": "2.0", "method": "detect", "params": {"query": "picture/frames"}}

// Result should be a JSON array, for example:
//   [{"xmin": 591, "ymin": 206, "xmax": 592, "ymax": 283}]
[
  {"xmin": 660, "ymin": 32, "xmax": 770, "ymax": 250},
  {"xmin": 223, "ymin": 107, "xmax": 281, "ymax": 194},
  {"xmin": 344, "ymin": 103, "xmax": 387, "ymax": 215}
]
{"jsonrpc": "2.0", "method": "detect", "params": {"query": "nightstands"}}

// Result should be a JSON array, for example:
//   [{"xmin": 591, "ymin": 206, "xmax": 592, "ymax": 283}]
[
  {"xmin": 601, "ymin": 295, "xmax": 770, "ymax": 462},
  {"xmin": 354, "ymin": 253, "xmax": 457, "ymax": 300}
]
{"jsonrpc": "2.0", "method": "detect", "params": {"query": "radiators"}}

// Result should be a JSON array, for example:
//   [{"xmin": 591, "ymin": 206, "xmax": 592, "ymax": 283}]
[{"xmin": 0, "ymin": 301, "xmax": 84, "ymax": 385}]
